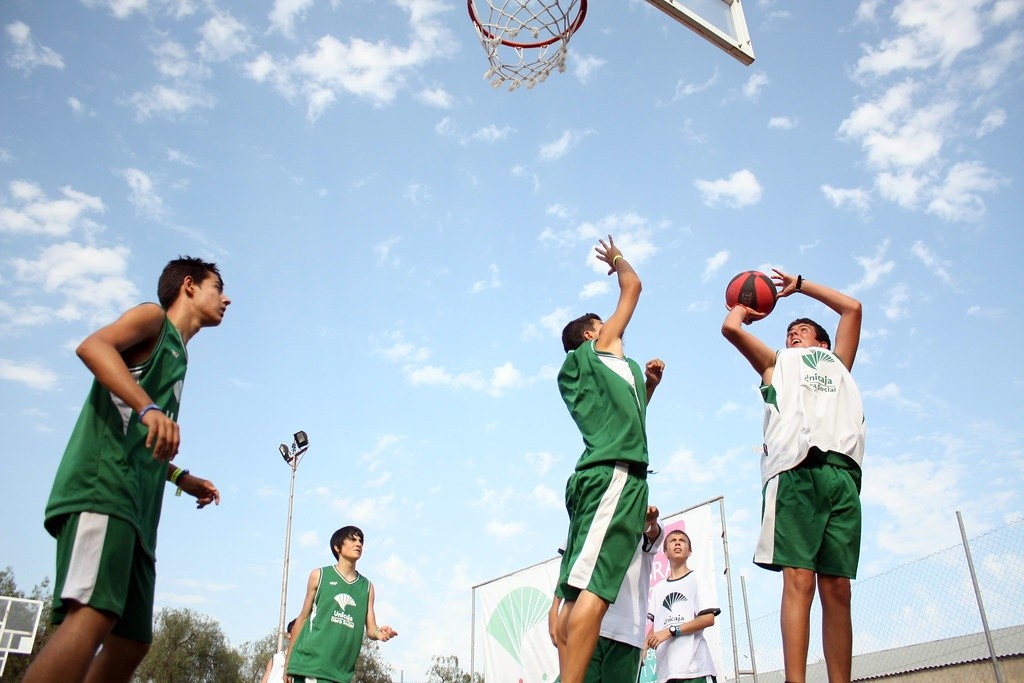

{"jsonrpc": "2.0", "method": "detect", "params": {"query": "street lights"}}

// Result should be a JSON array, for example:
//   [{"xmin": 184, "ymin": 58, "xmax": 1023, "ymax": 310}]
[{"xmin": 277, "ymin": 428, "xmax": 313, "ymax": 652}]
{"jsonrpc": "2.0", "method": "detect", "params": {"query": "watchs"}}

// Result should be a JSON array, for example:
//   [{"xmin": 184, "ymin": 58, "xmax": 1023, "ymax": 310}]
[{"xmin": 669, "ymin": 625, "xmax": 676, "ymax": 637}]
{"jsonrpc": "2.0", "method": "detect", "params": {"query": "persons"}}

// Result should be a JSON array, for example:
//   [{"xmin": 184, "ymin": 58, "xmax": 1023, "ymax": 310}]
[
  {"xmin": 282, "ymin": 526, "xmax": 398, "ymax": 683},
  {"xmin": 641, "ymin": 530, "xmax": 721, "ymax": 683},
  {"xmin": 721, "ymin": 267, "xmax": 866, "ymax": 683},
  {"xmin": 555, "ymin": 235, "xmax": 665, "ymax": 683},
  {"xmin": 21, "ymin": 254, "xmax": 231, "ymax": 683},
  {"xmin": 548, "ymin": 505, "xmax": 665, "ymax": 683},
  {"xmin": 262, "ymin": 619, "xmax": 317, "ymax": 683}
]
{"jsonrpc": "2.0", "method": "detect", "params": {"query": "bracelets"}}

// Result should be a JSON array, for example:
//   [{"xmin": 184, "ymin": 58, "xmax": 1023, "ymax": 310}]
[
  {"xmin": 733, "ymin": 303, "xmax": 749, "ymax": 318},
  {"xmin": 171, "ymin": 468, "xmax": 184, "ymax": 496},
  {"xmin": 795, "ymin": 275, "xmax": 805, "ymax": 292},
  {"xmin": 613, "ymin": 255, "xmax": 623, "ymax": 268},
  {"xmin": 138, "ymin": 402, "xmax": 161, "ymax": 423},
  {"xmin": 176, "ymin": 470, "xmax": 189, "ymax": 486},
  {"xmin": 676, "ymin": 626, "xmax": 680, "ymax": 636}
]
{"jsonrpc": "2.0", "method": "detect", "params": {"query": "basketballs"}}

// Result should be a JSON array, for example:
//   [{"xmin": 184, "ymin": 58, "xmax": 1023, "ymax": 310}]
[{"xmin": 725, "ymin": 271, "xmax": 778, "ymax": 321}]
